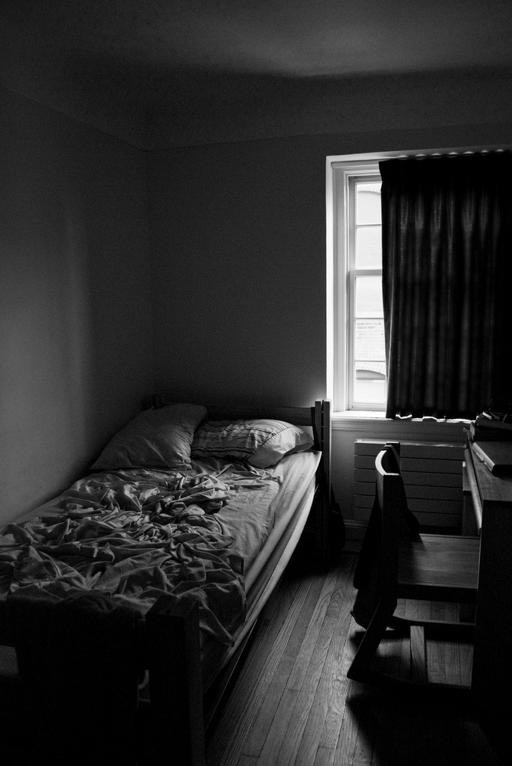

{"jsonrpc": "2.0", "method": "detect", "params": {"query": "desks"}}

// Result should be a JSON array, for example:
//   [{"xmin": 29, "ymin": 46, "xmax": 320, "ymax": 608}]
[{"xmin": 460, "ymin": 426, "xmax": 512, "ymax": 766}]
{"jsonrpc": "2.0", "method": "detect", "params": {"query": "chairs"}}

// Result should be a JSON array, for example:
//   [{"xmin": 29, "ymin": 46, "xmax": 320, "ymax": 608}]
[{"xmin": 347, "ymin": 440, "xmax": 481, "ymax": 687}]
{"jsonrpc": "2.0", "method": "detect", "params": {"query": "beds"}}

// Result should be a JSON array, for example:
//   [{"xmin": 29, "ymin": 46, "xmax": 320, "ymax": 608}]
[{"xmin": 0, "ymin": 396, "xmax": 329, "ymax": 766}]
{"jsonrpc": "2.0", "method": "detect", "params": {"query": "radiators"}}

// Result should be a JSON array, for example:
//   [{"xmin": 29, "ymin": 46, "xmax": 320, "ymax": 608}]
[{"xmin": 352, "ymin": 438, "xmax": 465, "ymax": 528}]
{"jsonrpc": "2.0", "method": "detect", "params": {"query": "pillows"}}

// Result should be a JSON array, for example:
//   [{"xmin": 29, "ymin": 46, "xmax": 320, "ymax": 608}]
[
  {"xmin": 89, "ymin": 403, "xmax": 206, "ymax": 471},
  {"xmin": 190, "ymin": 418, "xmax": 315, "ymax": 469}
]
